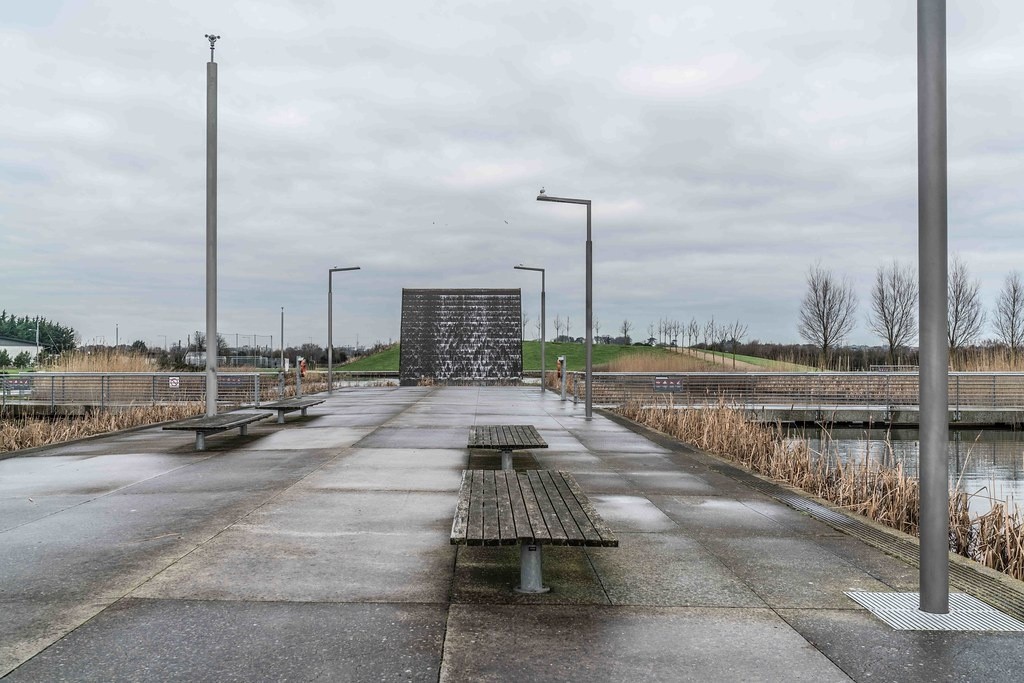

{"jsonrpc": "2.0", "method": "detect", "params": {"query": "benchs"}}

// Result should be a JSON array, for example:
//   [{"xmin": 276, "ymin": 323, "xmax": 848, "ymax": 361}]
[
  {"xmin": 449, "ymin": 470, "xmax": 619, "ymax": 594},
  {"xmin": 466, "ymin": 425, "xmax": 549, "ymax": 470},
  {"xmin": 162, "ymin": 413, "xmax": 272, "ymax": 452},
  {"xmin": 255, "ymin": 400, "xmax": 326, "ymax": 423}
]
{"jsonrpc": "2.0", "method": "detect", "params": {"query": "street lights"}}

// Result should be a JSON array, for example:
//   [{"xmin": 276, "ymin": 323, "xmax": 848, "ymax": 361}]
[
  {"xmin": 537, "ymin": 196, "xmax": 592, "ymax": 417},
  {"xmin": 514, "ymin": 266, "xmax": 545, "ymax": 394},
  {"xmin": 327, "ymin": 266, "xmax": 361, "ymax": 394}
]
